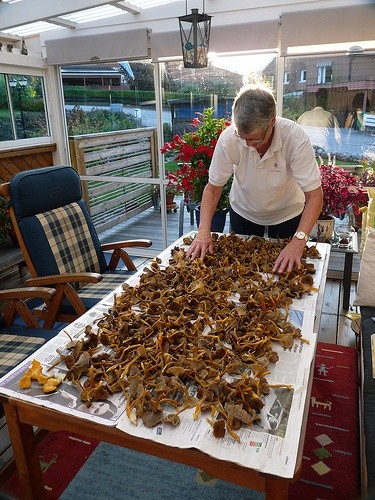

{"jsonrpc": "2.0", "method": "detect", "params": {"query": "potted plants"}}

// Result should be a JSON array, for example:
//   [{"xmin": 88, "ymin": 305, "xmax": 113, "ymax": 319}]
[{"xmin": 155, "ymin": 185, "xmax": 182, "ymax": 203}]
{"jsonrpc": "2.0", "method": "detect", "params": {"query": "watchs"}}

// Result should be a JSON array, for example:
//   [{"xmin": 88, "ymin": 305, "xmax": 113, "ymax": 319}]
[{"xmin": 293, "ymin": 231, "xmax": 308, "ymax": 243}]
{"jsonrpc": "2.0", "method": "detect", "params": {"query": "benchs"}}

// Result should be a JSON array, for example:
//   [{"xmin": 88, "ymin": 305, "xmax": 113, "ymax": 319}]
[{"xmin": 349, "ymin": 197, "xmax": 375, "ymax": 500}]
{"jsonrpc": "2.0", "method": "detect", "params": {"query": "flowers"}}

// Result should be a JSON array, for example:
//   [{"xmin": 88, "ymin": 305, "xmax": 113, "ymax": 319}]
[
  {"xmin": 160, "ymin": 108, "xmax": 234, "ymax": 208},
  {"xmin": 317, "ymin": 165, "xmax": 368, "ymax": 215}
]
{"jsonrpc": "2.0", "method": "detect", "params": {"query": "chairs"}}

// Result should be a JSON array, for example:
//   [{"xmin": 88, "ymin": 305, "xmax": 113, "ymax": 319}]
[
  {"xmin": 0, "ymin": 286, "xmax": 62, "ymax": 379},
  {"xmin": 0, "ymin": 164, "xmax": 152, "ymax": 322}
]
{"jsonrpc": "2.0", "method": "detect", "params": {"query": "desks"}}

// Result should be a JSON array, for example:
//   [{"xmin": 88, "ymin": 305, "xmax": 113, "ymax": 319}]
[
  {"xmin": 0, "ymin": 229, "xmax": 331, "ymax": 500},
  {"xmin": 330, "ymin": 232, "xmax": 358, "ymax": 311}
]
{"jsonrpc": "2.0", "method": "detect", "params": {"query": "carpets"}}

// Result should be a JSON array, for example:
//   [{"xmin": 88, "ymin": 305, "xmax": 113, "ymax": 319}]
[{"xmin": 1, "ymin": 340, "xmax": 358, "ymax": 500}]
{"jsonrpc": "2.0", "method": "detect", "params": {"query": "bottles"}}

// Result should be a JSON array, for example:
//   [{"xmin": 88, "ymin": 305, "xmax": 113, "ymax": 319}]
[{"xmin": 335, "ymin": 224, "xmax": 355, "ymax": 238}]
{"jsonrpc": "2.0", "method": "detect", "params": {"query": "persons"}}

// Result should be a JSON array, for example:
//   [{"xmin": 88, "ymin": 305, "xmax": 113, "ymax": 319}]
[
  {"xmin": 184, "ymin": 87, "xmax": 324, "ymax": 273},
  {"xmin": 296, "ymin": 94, "xmax": 342, "ymax": 153},
  {"xmin": 345, "ymin": 107, "xmax": 366, "ymax": 132}
]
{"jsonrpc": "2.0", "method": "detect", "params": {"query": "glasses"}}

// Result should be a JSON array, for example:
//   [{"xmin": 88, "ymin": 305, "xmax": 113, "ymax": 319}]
[{"xmin": 234, "ymin": 124, "xmax": 270, "ymax": 143}]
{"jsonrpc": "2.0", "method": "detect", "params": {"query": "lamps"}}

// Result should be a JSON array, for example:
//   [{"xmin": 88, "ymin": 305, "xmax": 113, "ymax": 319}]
[{"xmin": 178, "ymin": 0, "xmax": 212, "ymax": 68}]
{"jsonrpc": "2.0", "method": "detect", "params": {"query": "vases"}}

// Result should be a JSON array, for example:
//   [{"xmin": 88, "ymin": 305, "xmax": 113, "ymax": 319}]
[
  {"xmin": 307, "ymin": 214, "xmax": 335, "ymax": 244},
  {"xmin": 195, "ymin": 204, "xmax": 228, "ymax": 233}
]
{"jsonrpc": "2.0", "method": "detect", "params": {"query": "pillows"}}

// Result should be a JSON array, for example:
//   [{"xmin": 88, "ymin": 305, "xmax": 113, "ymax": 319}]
[{"xmin": 354, "ymin": 225, "xmax": 375, "ymax": 307}]
